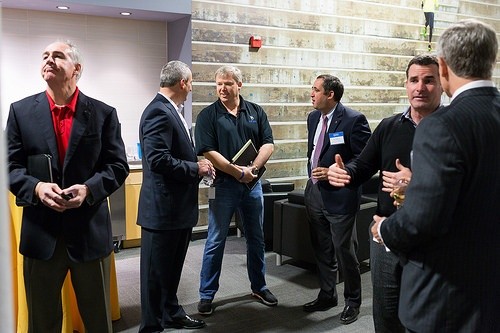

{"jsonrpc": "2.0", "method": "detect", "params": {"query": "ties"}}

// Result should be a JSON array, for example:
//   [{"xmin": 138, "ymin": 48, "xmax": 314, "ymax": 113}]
[{"xmin": 311, "ymin": 114, "xmax": 327, "ymax": 185}]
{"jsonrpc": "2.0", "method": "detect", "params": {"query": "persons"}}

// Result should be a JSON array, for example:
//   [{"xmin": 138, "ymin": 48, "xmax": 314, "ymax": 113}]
[
  {"xmin": 135, "ymin": 60, "xmax": 218, "ymax": 333},
  {"xmin": 194, "ymin": 64, "xmax": 279, "ymax": 315},
  {"xmin": 303, "ymin": 74, "xmax": 372, "ymax": 324},
  {"xmin": 326, "ymin": 19, "xmax": 500, "ymax": 333},
  {"xmin": 420, "ymin": 0, "xmax": 441, "ymax": 52},
  {"xmin": 4, "ymin": 38, "xmax": 131, "ymax": 333}
]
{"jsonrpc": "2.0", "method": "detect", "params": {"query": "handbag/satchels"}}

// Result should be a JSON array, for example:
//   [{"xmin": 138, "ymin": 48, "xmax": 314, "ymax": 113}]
[{"xmin": 16, "ymin": 154, "xmax": 53, "ymax": 206}]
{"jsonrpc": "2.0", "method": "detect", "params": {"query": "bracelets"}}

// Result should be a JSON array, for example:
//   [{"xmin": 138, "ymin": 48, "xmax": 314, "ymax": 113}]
[{"xmin": 236, "ymin": 168, "xmax": 245, "ymax": 182}]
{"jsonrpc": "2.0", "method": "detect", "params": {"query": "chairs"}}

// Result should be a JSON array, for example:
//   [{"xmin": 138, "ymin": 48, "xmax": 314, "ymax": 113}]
[
  {"xmin": 271, "ymin": 190, "xmax": 377, "ymax": 284},
  {"xmin": 237, "ymin": 181, "xmax": 295, "ymax": 251}
]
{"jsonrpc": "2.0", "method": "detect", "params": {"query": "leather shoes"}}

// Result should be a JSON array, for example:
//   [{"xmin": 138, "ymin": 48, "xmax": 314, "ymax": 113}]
[
  {"xmin": 303, "ymin": 291, "xmax": 337, "ymax": 312},
  {"xmin": 340, "ymin": 305, "xmax": 360, "ymax": 325},
  {"xmin": 161, "ymin": 308, "xmax": 205, "ymax": 329}
]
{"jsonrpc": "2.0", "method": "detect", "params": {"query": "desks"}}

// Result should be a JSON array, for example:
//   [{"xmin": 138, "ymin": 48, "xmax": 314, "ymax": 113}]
[{"xmin": 123, "ymin": 162, "xmax": 144, "ymax": 249}]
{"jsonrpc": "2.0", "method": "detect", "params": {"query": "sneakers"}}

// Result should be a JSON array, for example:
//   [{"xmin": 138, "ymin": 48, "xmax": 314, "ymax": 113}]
[
  {"xmin": 252, "ymin": 289, "xmax": 278, "ymax": 305},
  {"xmin": 198, "ymin": 300, "xmax": 212, "ymax": 314}
]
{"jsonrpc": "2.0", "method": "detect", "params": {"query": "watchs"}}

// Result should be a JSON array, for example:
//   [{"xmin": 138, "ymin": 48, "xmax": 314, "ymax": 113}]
[{"xmin": 253, "ymin": 168, "xmax": 259, "ymax": 174}]
